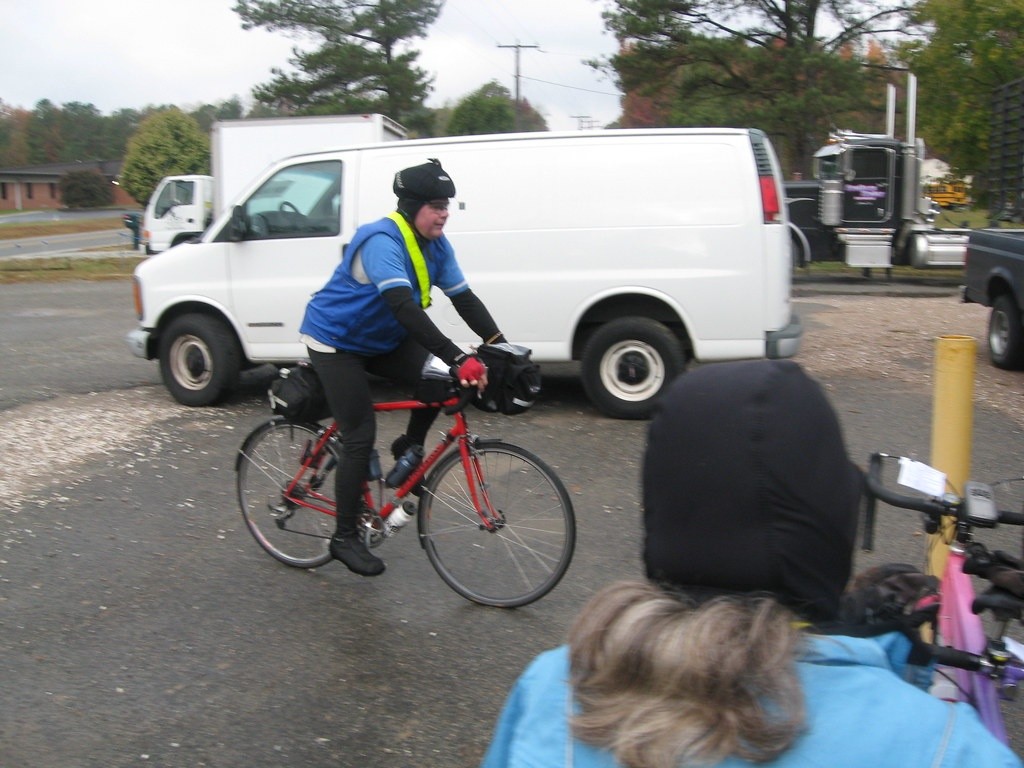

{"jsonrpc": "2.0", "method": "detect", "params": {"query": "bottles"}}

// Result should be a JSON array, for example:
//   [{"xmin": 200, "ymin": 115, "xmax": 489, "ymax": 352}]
[
  {"xmin": 385, "ymin": 445, "xmax": 424, "ymax": 486},
  {"xmin": 384, "ymin": 502, "xmax": 415, "ymax": 536}
]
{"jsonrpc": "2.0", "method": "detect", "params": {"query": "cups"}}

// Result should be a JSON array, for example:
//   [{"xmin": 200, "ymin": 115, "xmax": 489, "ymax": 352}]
[{"xmin": 369, "ymin": 450, "xmax": 381, "ymax": 481}]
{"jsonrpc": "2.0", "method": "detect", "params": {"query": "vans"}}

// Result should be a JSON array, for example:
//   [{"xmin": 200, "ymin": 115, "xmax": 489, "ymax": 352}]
[{"xmin": 125, "ymin": 126, "xmax": 803, "ymax": 406}]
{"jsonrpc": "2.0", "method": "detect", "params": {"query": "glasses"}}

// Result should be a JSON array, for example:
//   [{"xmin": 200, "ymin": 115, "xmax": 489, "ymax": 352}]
[{"xmin": 424, "ymin": 199, "xmax": 450, "ymax": 213}]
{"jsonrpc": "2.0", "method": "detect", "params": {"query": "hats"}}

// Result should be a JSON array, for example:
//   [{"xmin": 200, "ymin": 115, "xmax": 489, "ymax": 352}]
[
  {"xmin": 643, "ymin": 358, "xmax": 863, "ymax": 616},
  {"xmin": 393, "ymin": 157, "xmax": 455, "ymax": 202}
]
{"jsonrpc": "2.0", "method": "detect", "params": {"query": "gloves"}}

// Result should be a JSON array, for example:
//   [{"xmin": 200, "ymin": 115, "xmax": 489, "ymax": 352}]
[
  {"xmin": 961, "ymin": 549, "xmax": 1024, "ymax": 620},
  {"xmin": 843, "ymin": 561, "xmax": 943, "ymax": 638}
]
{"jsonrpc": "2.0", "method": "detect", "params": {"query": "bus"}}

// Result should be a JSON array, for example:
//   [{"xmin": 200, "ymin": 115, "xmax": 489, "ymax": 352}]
[{"xmin": 922, "ymin": 180, "xmax": 967, "ymax": 208}]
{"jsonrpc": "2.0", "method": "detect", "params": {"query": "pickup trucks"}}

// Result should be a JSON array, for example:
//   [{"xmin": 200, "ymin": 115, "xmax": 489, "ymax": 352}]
[{"xmin": 956, "ymin": 227, "xmax": 1024, "ymax": 371}]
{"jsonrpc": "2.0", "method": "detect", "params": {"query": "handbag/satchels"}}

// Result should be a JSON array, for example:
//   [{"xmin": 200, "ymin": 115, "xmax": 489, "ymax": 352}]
[{"xmin": 267, "ymin": 361, "xmax": 331, "ymax": 425}]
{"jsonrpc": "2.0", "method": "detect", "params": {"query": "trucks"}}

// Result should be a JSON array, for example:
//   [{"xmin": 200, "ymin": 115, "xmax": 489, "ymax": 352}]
[
  {"xmin": 784, "ymin": 71, "xmax": 1023, "ymax": 275},
  {"xmin": 138, "ymin": 112, "xmax": 407, "ymax": 255}
]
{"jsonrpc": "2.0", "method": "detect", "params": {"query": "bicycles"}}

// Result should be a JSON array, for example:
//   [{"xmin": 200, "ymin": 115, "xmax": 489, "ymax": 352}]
[
  {"xmin": 235, "ymin": 346, "xmax": 577, "ymax": 608},
  {"xmin": 862, "ymin": 452, "xmax": 1024, "ymax": 703}
]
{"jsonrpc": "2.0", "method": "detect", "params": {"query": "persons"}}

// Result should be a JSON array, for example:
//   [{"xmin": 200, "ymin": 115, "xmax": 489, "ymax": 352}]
[
  {"xmin": 478, "ymin": 360, "xmax": 1024, "ymax": 768},
  {"xmin": 298, "ymin": 162, "xmax": 509, "ymax": 577}
]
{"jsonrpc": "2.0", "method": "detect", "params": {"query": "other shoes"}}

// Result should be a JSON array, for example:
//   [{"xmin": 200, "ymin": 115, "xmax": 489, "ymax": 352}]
[
  {"xmin": 330, "ymin": 531, "xmax": 387, "ymax": 577},
  {"xmin": 391, "ymin": 433, "xmax": 426, "ymax": 496}
]
